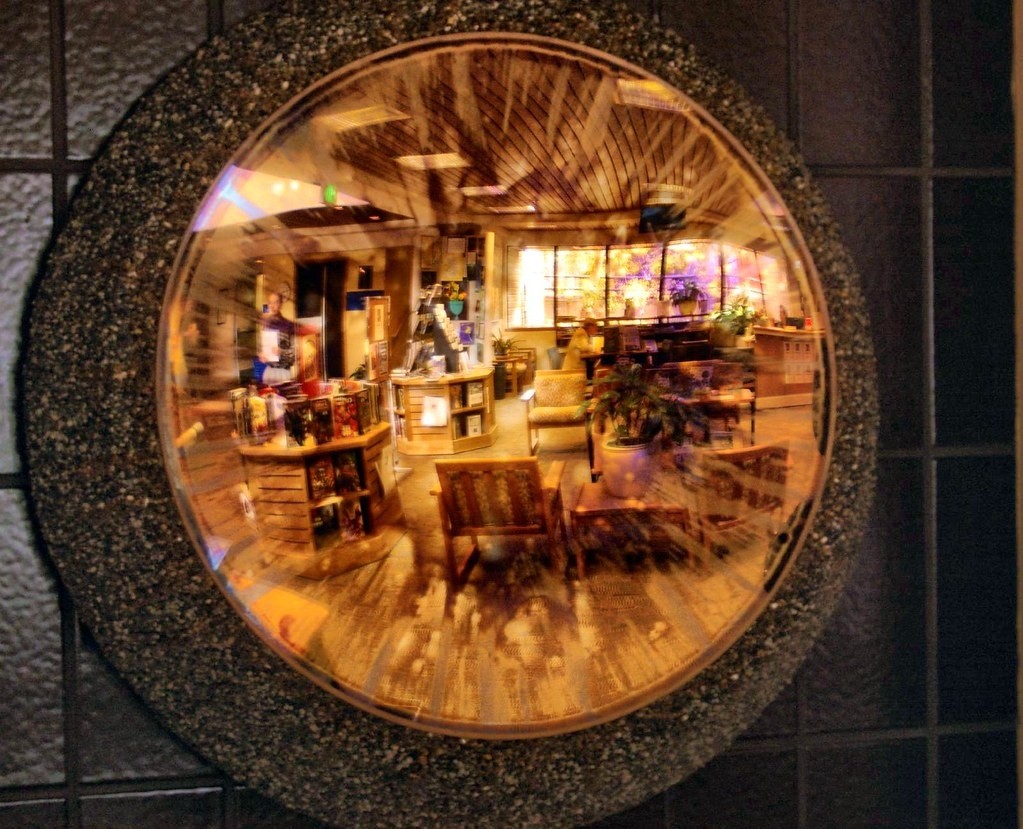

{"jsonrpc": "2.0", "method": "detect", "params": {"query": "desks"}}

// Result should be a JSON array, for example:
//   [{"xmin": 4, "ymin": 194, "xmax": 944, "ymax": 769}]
[
  {"xmin": 754, "ymin": 325, "xmax": 820, "ymax": 411},
  {"xmin": 230, "ymin": 422, "xmax": 408, "ymax": 580},
  {"xmin": 569, "ymin": 482, "xmax": 697, "ymax": 582},
  {"xmin": 387, "ymin": 365, "xmax": 496, "ymax": 457}
]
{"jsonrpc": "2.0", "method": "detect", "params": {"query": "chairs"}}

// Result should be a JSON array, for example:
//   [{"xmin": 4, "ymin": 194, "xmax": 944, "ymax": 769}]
[
  {"xmin": 429, "ymin": 456, "xmax": 567, "ymax": 593},
  {"xmin": 519, "ymin": 369, "xmax": 594, "ymax": 458},
  {"xmin": 674, "ymin": 439, "xmax": 789, "ymax": 574},
  {"xmin": 487, "ymin": 344, "xmax": 536, "ymax": 398}
]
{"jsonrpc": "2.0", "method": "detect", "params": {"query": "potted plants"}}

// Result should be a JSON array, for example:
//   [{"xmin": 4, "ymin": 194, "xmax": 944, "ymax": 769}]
[
  {"xmin": 574, "ymin": 366, "xmax": 708, "ymax": 497},
  {"xmin": 668, "ymin": 278, "xmax": 704, "ymax": 315}
]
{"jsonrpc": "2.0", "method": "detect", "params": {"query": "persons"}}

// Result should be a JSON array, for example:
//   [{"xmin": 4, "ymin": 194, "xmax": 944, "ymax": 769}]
[
  {"xmin": 562, "ymin": 317, "xmax": 597, "ymax": 380},
  {"xmin": 258, "ymin": 293, "xmax": 296, "ymax": 383}
]
